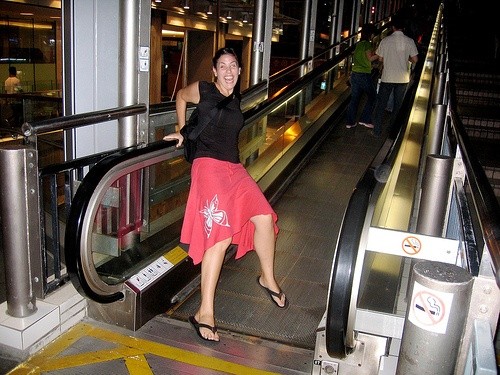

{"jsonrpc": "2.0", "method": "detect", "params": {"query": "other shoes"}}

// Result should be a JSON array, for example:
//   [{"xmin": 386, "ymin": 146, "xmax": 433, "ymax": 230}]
[
  {"xmin": 358, "ymin": 122, "xmax": 375, "ymax": 129},
  {"xmin": 346, "ymin": 122, "xmax": 358, "ymax": 129}
]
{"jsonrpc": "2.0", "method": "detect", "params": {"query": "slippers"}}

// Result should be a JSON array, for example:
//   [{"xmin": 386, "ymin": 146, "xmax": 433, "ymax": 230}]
[
  {"xmin": 257, "ymin": 275, "xmax": 289, "ymax": 310},
  {"xmin": 189, "ymin": 316, "xmax": 221, "ymax": 343}
]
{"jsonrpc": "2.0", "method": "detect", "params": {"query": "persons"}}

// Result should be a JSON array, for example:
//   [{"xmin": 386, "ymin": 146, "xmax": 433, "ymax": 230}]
[
  {"xmin": 367, "ymin": 18, "xmax": 418, "ymax": 137},
  {"xmin": 4, "ymin": 67, "xmax": 24, "ymax": 127},
  {"xmin": 368, "ymin": 0, "xmax": 435, "ymax": 116},
  {"xmin": 345, "ymin": 23, "xmax": 379, "ymax": 129},
  {"xmin": 164, "ymin": 47, "xmax": 289, "ymax": 346}
]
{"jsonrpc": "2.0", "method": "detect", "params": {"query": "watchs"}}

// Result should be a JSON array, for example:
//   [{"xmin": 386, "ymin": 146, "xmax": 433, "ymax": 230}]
[{"xmin": 175, "ymin": 124, "xmax": 183, "ymax": 132}]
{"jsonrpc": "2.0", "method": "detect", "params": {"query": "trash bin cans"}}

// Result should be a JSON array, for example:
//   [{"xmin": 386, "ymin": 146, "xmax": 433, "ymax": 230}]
[{"xmin": 396, "ymin": 261, "xmax": 474, "ymax": 374}]
